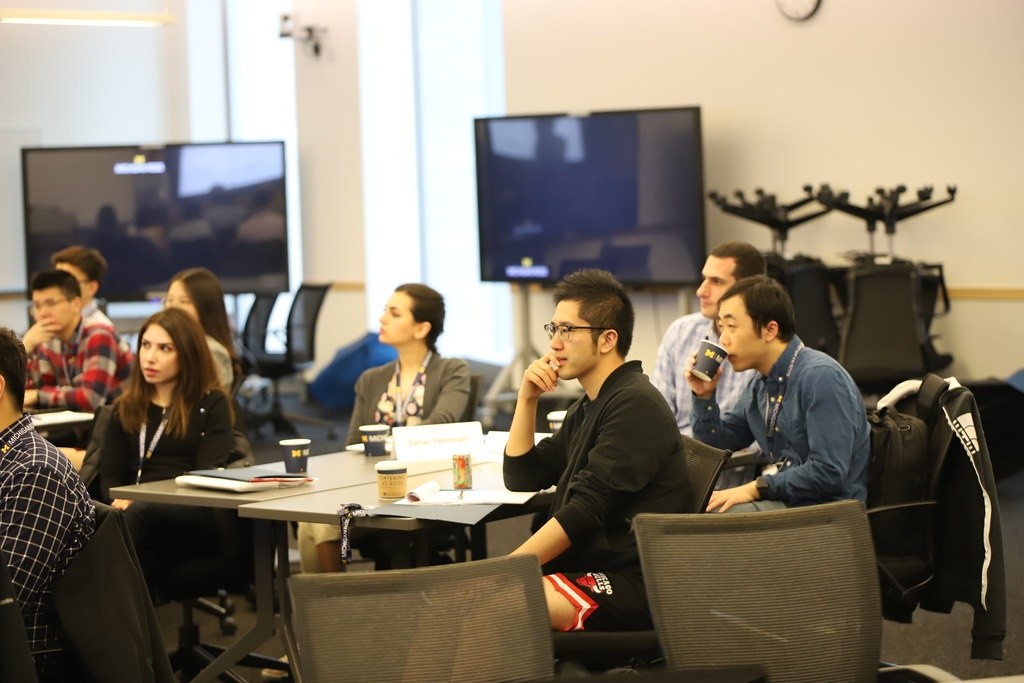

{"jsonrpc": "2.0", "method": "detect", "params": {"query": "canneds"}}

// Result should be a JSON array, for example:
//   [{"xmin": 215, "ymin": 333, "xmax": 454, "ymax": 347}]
[{"xmin": 452, "ymin": 453, "xmax": 472, "ymax": 489}]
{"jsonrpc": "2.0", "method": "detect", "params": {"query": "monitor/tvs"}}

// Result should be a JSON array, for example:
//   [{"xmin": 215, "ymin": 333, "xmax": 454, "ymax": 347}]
[
  {"xmin": 22, "ymin": 141, "xmax": 290, "ymax": 301},
  {"xmin": 473, "ymin": 106, "xmax": 707, "ymax": 284}
]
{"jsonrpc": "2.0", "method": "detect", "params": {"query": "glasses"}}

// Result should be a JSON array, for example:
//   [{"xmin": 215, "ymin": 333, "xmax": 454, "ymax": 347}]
[
  {"xmin": 30, "ymin": 297, "xmax": 73, "ymax": 310},
  {"xmin": 161, "ymin": 296, "xmax": 191, "ymax": 310},
  {"xmin": 544, "ymin": 324, "xmax": 613, "ymax": 341}
]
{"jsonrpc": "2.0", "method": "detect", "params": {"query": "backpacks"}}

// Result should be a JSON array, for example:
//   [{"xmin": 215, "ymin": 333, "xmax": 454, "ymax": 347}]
[{"xmin": 867, "ymin": 372, "xmax": 950, "ymax": 555}]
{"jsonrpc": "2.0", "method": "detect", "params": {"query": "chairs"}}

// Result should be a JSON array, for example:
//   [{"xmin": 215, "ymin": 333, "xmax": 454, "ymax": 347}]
[
  {"xmin": 231, "ymin": 282, "xmax": 330, "ymax": 435},
  {"xmin": 288, "ymin": 179, "xmax": 980, "ymax": 682}
]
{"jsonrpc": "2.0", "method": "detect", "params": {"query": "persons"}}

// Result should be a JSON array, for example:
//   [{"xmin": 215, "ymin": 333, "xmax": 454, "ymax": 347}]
[
  {"xmin": 0, "ymin": 327, "xmax": 97, "ymax": 683},
  {"xmin": 164, "ymin": 267, "xmax": 242, "ymax": 393},
  {"xmin": 650, "ymin": 242, "xmax": 767, "ymax": 492},
  {"xmin": 21, "ymin": 246, "xmax": 135, "ymax": 447},
  {"xmin": 685, "ymin": 275, "xmax": 872, "ymax": 514},
  {"xmin": 260, "ymin": 283, "xmax": 471, "ymax": 678},
  {"xmin": 502, "ymin": 269, "xmax": 695, "ymax": 632},
  {"xmin": 98, "ymin": 307, "xmax": 234, "ymax": 553}
]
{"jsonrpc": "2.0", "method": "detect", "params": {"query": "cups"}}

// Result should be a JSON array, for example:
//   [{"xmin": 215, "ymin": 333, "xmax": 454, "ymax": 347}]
[
  {"xmin": 547, "ymin": 410, "xmax": 569, "ymax": 432},
  {"xmin": 279, "ymin": 439, "xmax": 312, "ymax": 474},
  {"xmin": 690, "ymin": 339, "xmax": 727, "ymax": 381},
  {"xmin": 359, "ymin": 424, "xmax": 389, "ymax": 456},
  {"xmin": 373, "ymin": 460, "xmax": 407, "ymax": 501}
]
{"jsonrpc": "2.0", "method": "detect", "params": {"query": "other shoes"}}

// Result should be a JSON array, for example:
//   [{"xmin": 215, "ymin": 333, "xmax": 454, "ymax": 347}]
[{"xmin": 261, "ymin": 654, "xmax": 288, "ymax": 678}]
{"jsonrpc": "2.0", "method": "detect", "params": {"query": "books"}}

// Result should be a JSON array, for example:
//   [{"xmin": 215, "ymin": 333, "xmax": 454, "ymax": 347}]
[{"xmin": 175, "ymin": 467, "xmax": 314, "ymax": 492}]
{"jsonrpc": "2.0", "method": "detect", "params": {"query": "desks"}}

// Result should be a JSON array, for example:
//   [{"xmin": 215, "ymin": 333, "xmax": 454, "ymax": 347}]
[
  {"xmin": 109, "ymin": 446, "xmax": 394, "ymax": 683},
  {"xmin": 28, "ymin": 404, "xmax": 96, "ymax": 446},
  {"xmin": 237, "ymin": 447, "xmax": 759, "ymax": 683},
  {"xmin": 108, "ymin": 316, "xmax": 151, "ymax": 346}
]
